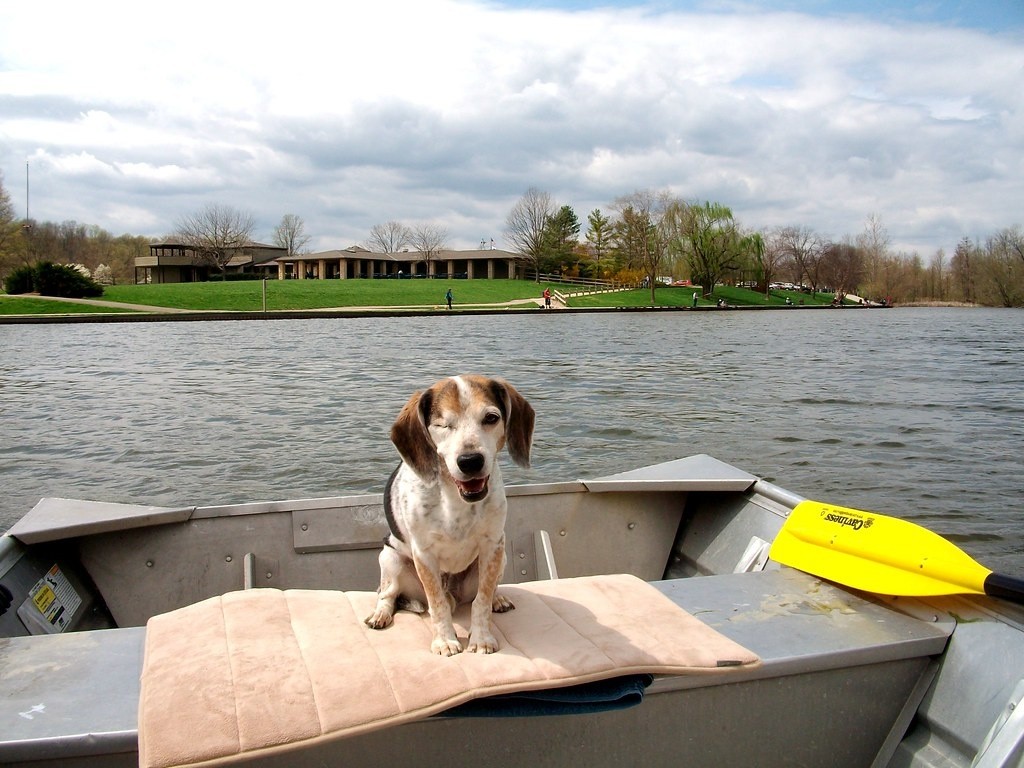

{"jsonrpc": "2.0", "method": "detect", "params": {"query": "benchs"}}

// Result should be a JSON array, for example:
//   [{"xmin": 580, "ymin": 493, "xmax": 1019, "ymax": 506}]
[{"xmin": 0, "ymin": 566, "xmax": 959, "ymax": 768}]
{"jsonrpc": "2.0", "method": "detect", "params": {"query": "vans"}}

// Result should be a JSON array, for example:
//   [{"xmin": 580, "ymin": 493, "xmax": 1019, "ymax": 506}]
[{"xmin": 646, "ymin": 276, "xmax": 673, "ymax": 285}]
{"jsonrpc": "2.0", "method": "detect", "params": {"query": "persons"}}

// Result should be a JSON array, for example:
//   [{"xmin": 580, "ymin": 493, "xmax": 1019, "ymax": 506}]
[
  {"xmin": 786, "ymin": 297, "xmax": 793, "ymax": 305},
  {"xmin": 544, "ymin": 288, "xmax": 551, "ymax": 309},
  {"xmin": 864, "ymin": 297, "xmax": 869, "ymax": 304},
  {"xmin": 305, "ymin": 272, "xmax": 312, "ymax": 279},
  {"xmin": 880, "ymin": 297, "xmax": 886, "ymax": 305},
  {"xmin": 840, "ymin": 299, "xmax": 846, "ymax": 307},
  {"xmin": 717, "ymin": 299, "xmax": 728, "ymax": 308},
  {"xmin": 832, "ymin": 298, "xmax": 838, "ymax": 305},
  {"xmin": 692, "ymin": 291, "xmax": 697, "ymax": 307},
  {"xmin": 859, "ymin": 299, "xmax": 862, "ymax": 305},
  {"xmin": 446, "ymin": 289, "xmax": 453, "ymax": 309}
]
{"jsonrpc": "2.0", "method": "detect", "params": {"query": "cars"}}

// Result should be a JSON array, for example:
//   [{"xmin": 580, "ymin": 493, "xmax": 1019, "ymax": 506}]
[
  {"xmin": 769, "ymin": 281, "xmax": 812, "ymax": 294},
  {"xmin": 674, "ymin": 280, "xmax": 690, "ymax": 285}
]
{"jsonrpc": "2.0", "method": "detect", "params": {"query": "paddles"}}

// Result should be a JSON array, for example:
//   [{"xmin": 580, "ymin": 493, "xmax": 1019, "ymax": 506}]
[{"xmin": 765, "ymin": 497, "xmax": 1024, "ymax": 609}]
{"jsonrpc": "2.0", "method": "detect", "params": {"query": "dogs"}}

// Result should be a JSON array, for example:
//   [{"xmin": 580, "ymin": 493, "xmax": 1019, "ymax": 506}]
[{"xmin": 364, "ymin": 374, "xmax": 535, "ymax": 658}]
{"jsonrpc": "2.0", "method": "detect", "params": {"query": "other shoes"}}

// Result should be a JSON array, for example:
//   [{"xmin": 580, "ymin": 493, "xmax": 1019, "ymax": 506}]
[
  {"xmin": 549, "ymin": 307, "xmax": 551, "ymax": 308},
  {"xmin": 546, "ymin": 307, "xmax": 547, "ymax": 308}
]
